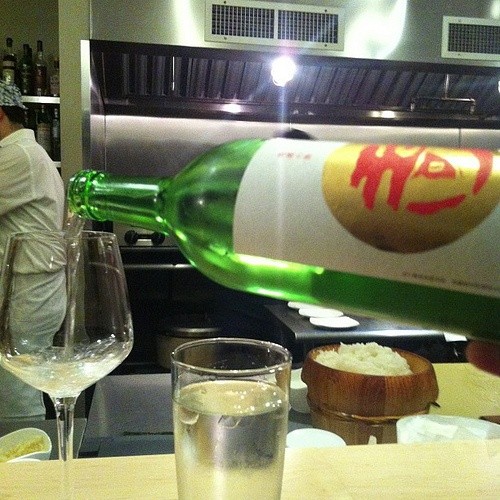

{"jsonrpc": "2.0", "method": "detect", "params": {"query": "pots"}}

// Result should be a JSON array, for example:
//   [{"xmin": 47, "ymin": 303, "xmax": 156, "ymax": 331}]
[{"xmin": 157, "ymin": 308, "xmax": 226, "ymax": 368}]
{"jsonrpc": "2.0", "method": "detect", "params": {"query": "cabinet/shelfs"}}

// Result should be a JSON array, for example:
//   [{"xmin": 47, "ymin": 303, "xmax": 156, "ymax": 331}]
[{"xmin": 20, "ymin": 95, "xmax": 61, "ymax": 169}]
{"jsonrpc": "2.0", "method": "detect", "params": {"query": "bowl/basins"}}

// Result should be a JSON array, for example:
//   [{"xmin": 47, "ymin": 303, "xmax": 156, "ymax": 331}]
[
  {"xmin": 302, "ymin": 344, "xmax": 438, "ymax": 416},
  {"xmin": 0, "ymin": 428, "xmax": 52, "ymax": 460}
]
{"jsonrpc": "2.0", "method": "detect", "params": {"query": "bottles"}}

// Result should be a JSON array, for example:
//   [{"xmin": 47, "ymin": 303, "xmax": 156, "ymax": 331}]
[
  {"xmin": 0, "ymin": 37, "xmax": 34, "ymax": 96},
  {"xmin": 32, "ymin": 40, "xmax": 49, "ymax": 95},
  {"xmin": 51, "ymin": 108, "xmax": 61, "ymax": 161},
  {"xmin": 23, "ymin": 104, "xmax": 52, "ymax": 158},
  {"xmin": 68, "ymin": 139, "xmax": 500, "ymax": 346},
  {"xmin": 49, "ymin": 58, "xmax": 60, "ymax": 96}
]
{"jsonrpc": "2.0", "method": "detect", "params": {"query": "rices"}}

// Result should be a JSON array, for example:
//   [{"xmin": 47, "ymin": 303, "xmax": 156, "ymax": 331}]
[{"xmin": 313, "ymin": 342, "xmax": 413, "ymax": 375}]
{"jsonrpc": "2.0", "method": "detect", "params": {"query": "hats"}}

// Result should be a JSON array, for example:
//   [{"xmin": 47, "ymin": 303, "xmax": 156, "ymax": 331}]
[{"xmin": 0, "ymin": 80, "xmax": 28, "ymax": 111}]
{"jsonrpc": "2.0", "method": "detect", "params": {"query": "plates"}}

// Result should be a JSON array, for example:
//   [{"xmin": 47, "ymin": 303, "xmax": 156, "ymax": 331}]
[
  {"xmin": 288, "ymin": 300, "xmax": 312, "ymax": 309},
  {"xmin": 309, "ymin": 315, "xmax": 360, "ymax": 329},
  {"xmin": 298, "ymin": 307, "xmax": 345, "ymax": 317}
]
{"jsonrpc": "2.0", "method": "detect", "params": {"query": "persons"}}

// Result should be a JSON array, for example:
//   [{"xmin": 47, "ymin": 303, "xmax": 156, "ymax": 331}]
[{"xmin": 0, "ymin": 76, "xmax": 65, "ymax": 422}]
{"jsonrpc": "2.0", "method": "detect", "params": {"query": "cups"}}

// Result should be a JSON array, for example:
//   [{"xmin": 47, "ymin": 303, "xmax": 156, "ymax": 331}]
[
  {"xmin": 393, "ymin": 415, "xmax": 500, "ymax": 446},
  {"xmin": 307, "ymin": 393, "xmax": 433, "ymax": 446},
  {"xmin": 287, "ymin": 428, "xmax": 347, "ymax": 447},
  {"xmin": 172, "ymin": 338, "xmax": 296, "ymax": 498},
  {"xmin": 289, "ymin": 368, "xmax": 311, "ymax": 415}
]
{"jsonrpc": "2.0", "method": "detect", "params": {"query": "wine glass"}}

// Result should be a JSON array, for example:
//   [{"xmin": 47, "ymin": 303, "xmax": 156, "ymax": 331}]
[{"xmin": 0, "ymin": 230, "xmax": 134, "ymax": 462}]
